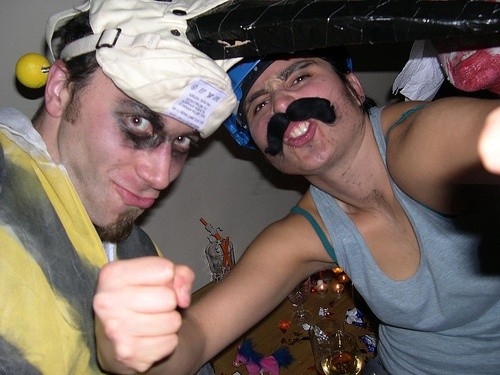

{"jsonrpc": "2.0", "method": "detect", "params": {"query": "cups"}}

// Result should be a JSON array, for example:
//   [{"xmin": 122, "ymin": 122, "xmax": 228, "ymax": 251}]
[
  {"xmin": 312, "ymin": 319, "xmax": 344, "ymax": 375},
  {"xmin": 204, "ymin": 238, "xmax": 235, "ymax": 284}
]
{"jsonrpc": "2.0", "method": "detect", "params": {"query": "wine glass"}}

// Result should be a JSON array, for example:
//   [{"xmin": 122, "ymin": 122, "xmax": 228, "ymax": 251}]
[
  {"xmin": 286, "ymin": 276, "xmax": 315, "ymax": 324},
  {"xmin": 321, "ymin": 329, "xmax": 362, "ymax": 375}
]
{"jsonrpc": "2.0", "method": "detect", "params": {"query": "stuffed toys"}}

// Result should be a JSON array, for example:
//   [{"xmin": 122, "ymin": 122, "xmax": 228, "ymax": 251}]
[{"xmin": 232, "ymin": 339, "xmax": 294, "ymax": 374}]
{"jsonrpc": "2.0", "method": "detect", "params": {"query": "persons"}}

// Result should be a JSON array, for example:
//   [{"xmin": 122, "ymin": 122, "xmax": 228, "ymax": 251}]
[
  {"xmin": 0, "ymin": 0, "xmax": 237, "ymax": 375},
  {"xmin": 437, "ymin": 50, "xmax": 500, "ymax": 95},
  {"xmin": 91, "ymin": 48, "xmax": 500, "ymax": 375}
]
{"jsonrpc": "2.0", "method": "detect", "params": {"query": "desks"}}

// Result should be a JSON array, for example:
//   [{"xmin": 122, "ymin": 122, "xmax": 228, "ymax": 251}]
[{"xmin": 176, "ymin": 269, "xmax": 380, "ymax": 375}]
